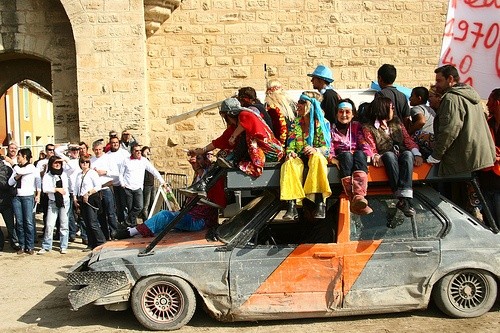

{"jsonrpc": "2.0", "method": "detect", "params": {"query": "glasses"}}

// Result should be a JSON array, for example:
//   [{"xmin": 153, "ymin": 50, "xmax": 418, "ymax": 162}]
[
  {"xmin": 390, "ymin": 106, "xmax": 396, "ymax": 112},
  {"xmin": 337, "ymin": 109, "xmax": 352, "ymax": 116},
  {"xmin": 298, "ymin": 100, "xmax": 309, "ymax": 105},
  {"xmin": 134, "ymin": 149, "xmax": 142, "ymax": 153},
  {"xmin": 80, "ymin": 159, "xmax": 90, "ymax": 163},
  {"xmin": 110, "ymin": 135, "xmax": 117, "ymax": 137},
  {"xmin": 47, "ymin": 149, "xmax": 54, "ymax": 152}
]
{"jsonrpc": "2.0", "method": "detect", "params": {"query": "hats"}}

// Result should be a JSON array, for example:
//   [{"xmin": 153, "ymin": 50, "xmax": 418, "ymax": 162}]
[
  {"xmin": 222, "ymin": 98, "xmax": 241, "ymax": 113},
  {"xmin": 306, "ymin": 65, "xmax": 334, "ymax": 83}
]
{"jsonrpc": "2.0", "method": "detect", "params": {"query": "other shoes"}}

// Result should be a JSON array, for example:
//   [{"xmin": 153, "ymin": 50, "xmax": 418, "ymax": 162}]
[
  {"xmin": 69, "ymin": 238, "xmax": 75, "ymax": 242},
  {"xmin": 17, "ymin": 247, "xmax": 24, "ymax": 254},
  {"xmin": 38, "ymin": 233, "xmax": 45, "ymax": 238},
  {"xmin": 113, "ymin": 229, "xmax": 135, "ymax": 239},
  {"xmin": 75, "ymin": 231, "xmax": 81, "ymax": 237},
  {"xmin": 217, "ymin": 157, "xmax": 233, "ymax": 169},
  {"xmin": 83, "ymin": 246, "xmax": 93, "ymax": 251},
  {"xmin": 52, "ymin": 235, "xmax": 59, "ymax": 240},
  {"xmin": 37, "ymin": 248, "xmax": 48, "ymax": 254},
  {"xmin": 59, "ymin": 248, "xmax": 66, "ymax": 254},
  {"xmin": 82, "ymin": 238, "xmax": 88, "ymax": 245},
  {"xmin": 396, "ymin": 199, "xmax": 416, "ymax": 217},
  {"xmin": 24, "ymin": 247, "xmax": 34, "ymax": 255}
]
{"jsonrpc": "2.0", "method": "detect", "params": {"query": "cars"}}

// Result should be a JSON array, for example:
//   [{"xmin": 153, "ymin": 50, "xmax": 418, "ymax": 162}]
[{"xmin": 64, "ymin": 154, "xmax": 500, "ymax": 331}]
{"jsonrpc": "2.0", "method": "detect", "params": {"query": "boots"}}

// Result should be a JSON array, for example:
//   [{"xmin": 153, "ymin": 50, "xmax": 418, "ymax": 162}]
[
  {"xmin": 341, "ymin": 176, "xmax": 373, "ymax": 215},
  {"xmin": 352, "ymin": 170, "xmax": 369, "ymax": 209},
  {"xmin": 282, "ymin": 200, "xmax": 298, "ymax": 221},
  {"xmin": 313, "ymin": 193, "xmax": 327, "ymax": 218}
]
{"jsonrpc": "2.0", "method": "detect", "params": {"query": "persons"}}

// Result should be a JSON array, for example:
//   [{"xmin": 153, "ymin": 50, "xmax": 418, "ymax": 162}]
[
  {"xmin": 280, "ymin": 91, "xmax": 332, "ymax": 220},
  {"xmin": 114, "ymin": 149, "xmax": 225, "ymax": 240},
  {"xmin": 37, "ymin": 156, "xmax": 73, "ymax": 254},
  {"xmin": 329, "ymin": 98, "xmax": 383, "ymax": 213},
  {"xmin": 0, "ymin": 130, "xmax": 169, "ymax": 256},
  {"xmin": 192, "ymin": 64, "xmax": 500, "ymax": 231},
  {"xmin": 427, "ymin": 65, "xmax": 496, "ymax": 177},
  {"xmin": 119, "ymin": 145, "xmax": 169, "ymax": 228},
  {"xmin": 73, "ymin": 156, "xmax": 106, "ymax": 252},
  {"xmin": 8, "ymin": 148, "xmax": 41, "ymax": 255}
]
